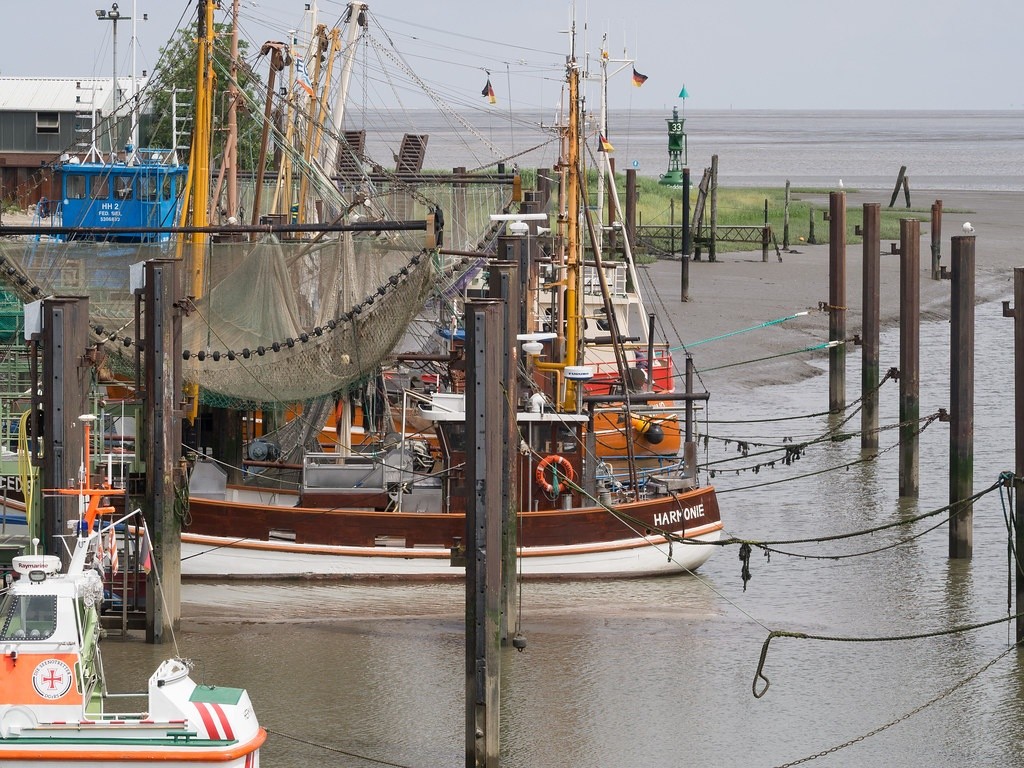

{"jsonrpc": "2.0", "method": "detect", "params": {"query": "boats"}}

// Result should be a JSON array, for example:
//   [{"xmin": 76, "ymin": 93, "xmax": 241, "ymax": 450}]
[{"xmin": 0, "ymin": 519, "xmax": 274, "ymax": 768}]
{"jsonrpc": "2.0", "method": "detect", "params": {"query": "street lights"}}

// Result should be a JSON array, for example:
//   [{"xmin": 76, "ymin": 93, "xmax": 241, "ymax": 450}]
[{"xmin": 94, "ymin": 3, "xmax": 132, "ymax": 166}]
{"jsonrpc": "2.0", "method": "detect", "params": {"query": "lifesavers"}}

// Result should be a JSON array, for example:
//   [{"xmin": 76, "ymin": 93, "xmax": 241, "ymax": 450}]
[
  {"xmin": 249, "ymin": 442, "xmax": 268, "ymax": 461},
  {"xmin": 535, "ymin": 455, "xmax": 574, "ymax": 493}
]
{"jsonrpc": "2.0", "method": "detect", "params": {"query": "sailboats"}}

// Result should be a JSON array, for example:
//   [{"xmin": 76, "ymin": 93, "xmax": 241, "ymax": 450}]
[{"xmin": 0, "ymin": 4, "xmax": 729, "ymax": 595}]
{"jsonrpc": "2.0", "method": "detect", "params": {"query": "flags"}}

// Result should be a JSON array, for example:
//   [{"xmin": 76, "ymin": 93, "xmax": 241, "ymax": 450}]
[
  {"xmin": 139, "ymin": 530, "xmax": 152, "ymax": 574},
  {"xmin": 482, "ymin": 81, "xmax": 496, "ymax": 103},
  {"xmin": 98, "ymin": 521, "xmax": 119, "ymax": 576},
  {"xmin": 631, "ymin": 69, "xmax": 648, "ymax": 87},
  {"xmin": 297, "ymin": 53, "xmax": 313, "ymax": 98},
  {"xmin": 597, "ymin": 135, "xmax": 614, "ymax": 152}
]
